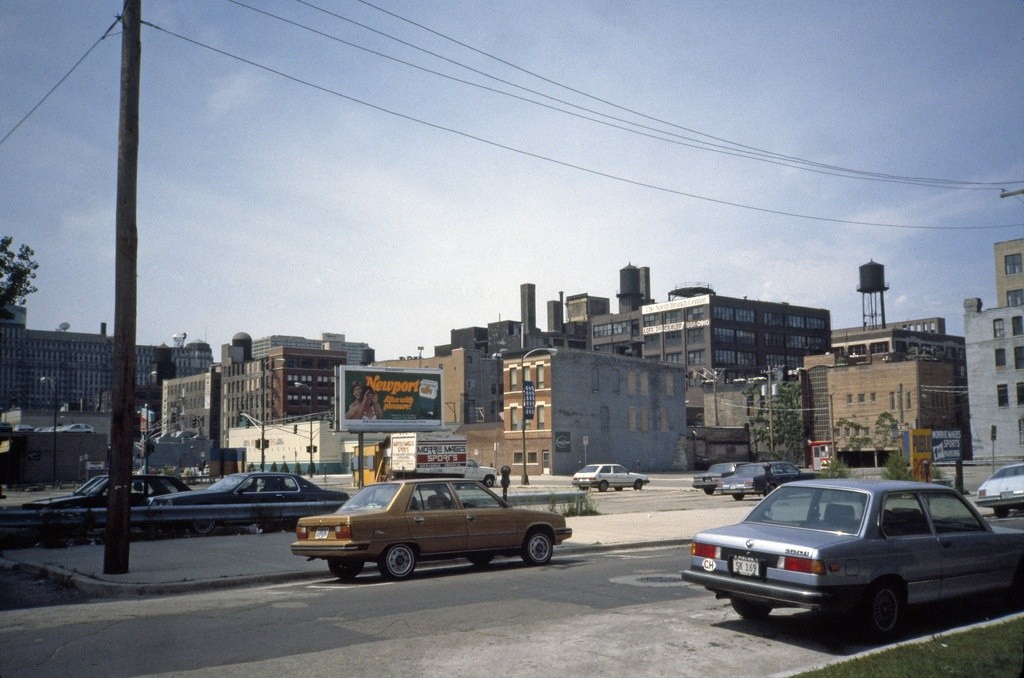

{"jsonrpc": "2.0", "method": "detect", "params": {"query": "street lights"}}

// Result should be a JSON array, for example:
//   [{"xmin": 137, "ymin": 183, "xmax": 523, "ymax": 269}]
[
  {"xmin": 296, "ymin": 382, "xmax": 314, "ymax": 477},
  {"xmin": 521, "ymin": 346, "xmax": 558, "ymax": 485},
  {"xmin": 144, "ymin": 371, "xmax": 157, "ymax": 473},
  {"xmin": 261, "ymin": 357, "xmax": 286, "ymax": 471},
  {"xmin": 40, "ymin": 376, "xmax": 59, "ymax": 487}
]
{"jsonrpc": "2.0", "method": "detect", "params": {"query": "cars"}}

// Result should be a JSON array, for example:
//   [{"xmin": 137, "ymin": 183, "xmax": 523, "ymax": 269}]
[
  {"xmin": 681, "ymin": 478, "xmax": 1024, "ymax": 638},
  {"xmin": 572, "ymin": 463, "xmax": 650, "ymax": 492},
  {"xmin": 291, "ymin": 476, "xmax": 572, "ymax": 580},
  {"xmin": 22, "ymin": 474, "xmax": 194, "ymax": 548},
  {"xmin": 974, "ymin": 463, "xmax": 1024, "ymax": 519},
  {"xmin": 716, "ymin": 460, "xmax": 818, "ymax": 500},
  {"xmin": 692, "ymin": 462, "xmax": 752, "ymax": 495},
  {"xmin": 0, "ymin": 420, "xmax": 95, "ymax": 433},
  {"xmin": 146, "ymin": 472, "xmax": 350, "ymax": 536}
]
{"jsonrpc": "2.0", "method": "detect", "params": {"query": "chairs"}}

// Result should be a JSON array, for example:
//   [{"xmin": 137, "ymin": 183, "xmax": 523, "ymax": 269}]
[
  {"xmin": 260, "ymin": 479, "xmax": 274, "ymax": 492},
  {"xmin": 882, "ymin": 507, "xmax": 926, "ymax": 534},
  {"xmin": 131, "ymin": 482, "xmax": 143, "ymax": 499},
  {"xmin": 823, "ymin": 503, "xmax": 855, "ymax": 532},
  {"xmin": 428, "ymin": 494, "xmax": 452, "ymax": 509},
  {"xmin": 407, "ymin": 496, "xmax": 419, "ymax": 510}
]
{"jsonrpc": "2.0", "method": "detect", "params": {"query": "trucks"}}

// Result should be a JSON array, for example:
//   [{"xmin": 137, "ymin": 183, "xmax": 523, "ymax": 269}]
[{"xmin": 389, "ymin": 434, "xmax": 497, "ymax": 487}]
